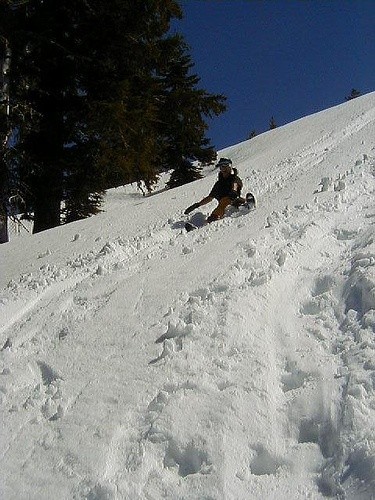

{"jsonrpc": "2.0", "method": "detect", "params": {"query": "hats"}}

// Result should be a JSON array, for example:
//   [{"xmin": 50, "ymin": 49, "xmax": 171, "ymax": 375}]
[{"xmin": 214, "ymin": 158, "xmax": 232, "ymax": 167}]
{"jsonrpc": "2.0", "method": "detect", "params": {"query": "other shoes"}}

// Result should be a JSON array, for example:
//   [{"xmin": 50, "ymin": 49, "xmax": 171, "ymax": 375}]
[
  {"xmin": 245, "ymin": 193, "xmax": 258, "ymax": 208},
  {"xmin": 183, "ymin": 222, "xmax": 198, "ymax": 231}
]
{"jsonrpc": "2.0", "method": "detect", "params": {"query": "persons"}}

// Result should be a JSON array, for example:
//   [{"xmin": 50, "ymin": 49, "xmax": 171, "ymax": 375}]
[{"xmin": 181, "ymin": 158, "xmax": 256, "ymax": 234}]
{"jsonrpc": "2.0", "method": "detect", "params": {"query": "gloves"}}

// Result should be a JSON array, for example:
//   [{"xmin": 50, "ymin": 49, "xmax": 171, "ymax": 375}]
[{"xmin": 184, "ymin": 203, "xmax": 200, "ymax": 215}]
